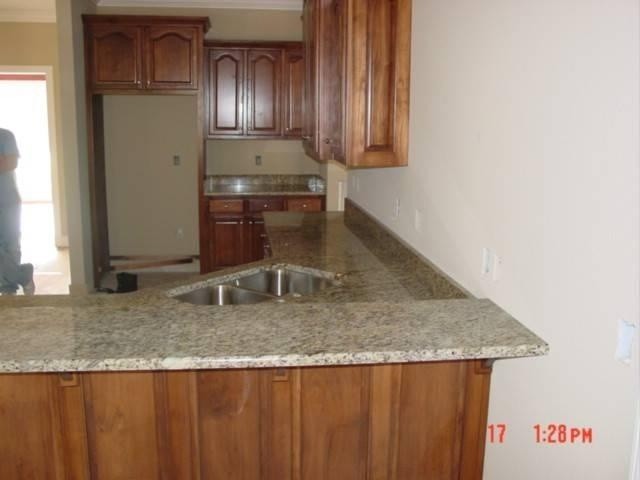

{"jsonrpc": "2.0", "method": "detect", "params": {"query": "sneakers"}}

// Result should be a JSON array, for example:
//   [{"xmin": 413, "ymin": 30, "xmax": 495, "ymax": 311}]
[{"xmin": 23, "ymin": 263, "xmax": 35, "ymax": 295}]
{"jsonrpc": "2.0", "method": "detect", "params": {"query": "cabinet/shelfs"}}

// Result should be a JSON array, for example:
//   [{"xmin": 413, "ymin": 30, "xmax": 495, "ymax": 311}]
[
  {"xmin": 198, "ymin": 193, "xmax": 325, "ymax": 274},
  {"xmin": 282, "ymin": 41, "xmax": 307, "ymax": 139},
  {"xmin": 301, "ymin": 0, "xmax": 412, "ymax": 169},
  {"xmin": 201, "ymin": 40, "xmax": 282, "ymax": 140},
  {"xmin": 81, "ymin": 13, "xmax": 211, "ymax": 95}
]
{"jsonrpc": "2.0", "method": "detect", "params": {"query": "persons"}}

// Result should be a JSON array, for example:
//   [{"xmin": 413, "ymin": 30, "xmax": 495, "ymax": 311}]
[{"xmin": 1, "ymin": 128, "xmax": 34, "ymax": 293}]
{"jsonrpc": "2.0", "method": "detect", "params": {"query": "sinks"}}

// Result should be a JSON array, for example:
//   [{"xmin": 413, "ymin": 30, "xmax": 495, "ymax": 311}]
[
  {"xmin": 227, "ymin": 269, "xmax": 336, "ymax": 297},
  {"xmin": 170, "ymin": 284, "xmax": 270, "ymax": 305}
]
